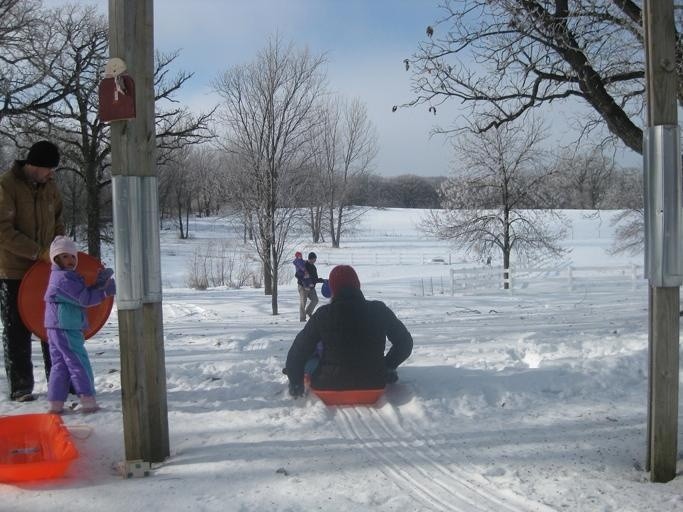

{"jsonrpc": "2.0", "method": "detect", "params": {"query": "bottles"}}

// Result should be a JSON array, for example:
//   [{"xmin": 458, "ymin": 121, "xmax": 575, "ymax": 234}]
[
  {"xmin": 27, "ymin": 141, "xmax": 60, "ymax": 167},
  {"xmin": 295, "ymin": 252, "xmax": 301, "ymax": 257},
  {"xmin": 329, "ymin": 265, "xmax": 360, "ymax": 294}
]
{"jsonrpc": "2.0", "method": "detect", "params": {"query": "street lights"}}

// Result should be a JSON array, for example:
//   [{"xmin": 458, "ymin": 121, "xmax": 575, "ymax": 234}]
[{"xmin": 321, "ymin": 280, "xmax": 331, "ymax": 298}]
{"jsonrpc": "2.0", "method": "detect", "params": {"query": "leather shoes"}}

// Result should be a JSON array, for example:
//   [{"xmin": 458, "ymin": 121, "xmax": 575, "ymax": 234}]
[
  {"xmin": 287, "ymin": 376, "xmax": 305, "ymax": 400},
  {"xmin": 97, "ymin": 268, "xmax": 116, "ymax": 297},
  {"xmin": 39, "ymin": 248, "xmax": 49, "ymax": 266},
  {"xmin": 384, "ymin": 368, "xmax": 398, "ymax": 385}
]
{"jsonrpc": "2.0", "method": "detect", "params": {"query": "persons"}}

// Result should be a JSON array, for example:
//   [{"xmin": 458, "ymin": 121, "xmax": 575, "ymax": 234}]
[
  {"xmin": 44, "ymin": 236, "xmax": 116, "ymax": 415},
  {"xmin": 293, "ymin": 251, "xmax": 314, "ymax": 291},
  {"xmin": 0, "ymin": 139, "xmax": 76, "ymax": 403},
  {"xmin": 284, "ymin": 264, "xmax": 413, "ymax": 399},
  {"xmin": 295, "ymin": 253, "xmax": 329, "ymax": 322}
]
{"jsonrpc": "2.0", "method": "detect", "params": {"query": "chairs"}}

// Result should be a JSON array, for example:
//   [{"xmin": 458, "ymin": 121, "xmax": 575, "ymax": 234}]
[
  {"xmin": 80, "ymin": 395, "xmax": 99, "ymax": 412},
  {"xmin": 11, "ymin": 394, "xmax": 32, "ymax": 401},
  {"xmin": 47, "ymin": 401, "xmax": 64, "ymax": 414}
]
